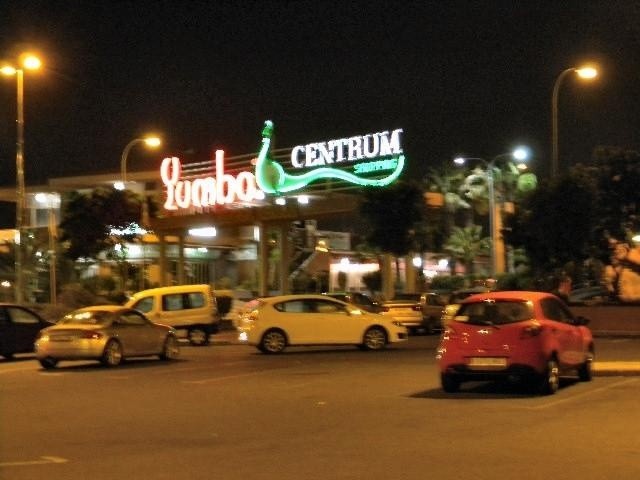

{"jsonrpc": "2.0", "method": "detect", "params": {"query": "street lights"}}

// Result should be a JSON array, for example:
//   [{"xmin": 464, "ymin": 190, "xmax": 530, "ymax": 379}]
[
  {"xmin": 119, "ymin": 135, "xmax": 162, "ymax": 183},
  {"xmin": 550, "ymin": 66, "xmax": 599, "ymax": 174},
  {"xmin": 453, "ymin": 148, "xmax": 527, "ymax": 275},
  {"xmin": 0, "ymin": 53, "xmax": 43, "ymax": 301}
]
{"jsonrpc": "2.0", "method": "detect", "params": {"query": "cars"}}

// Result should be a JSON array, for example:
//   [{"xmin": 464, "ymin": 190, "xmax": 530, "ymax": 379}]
[
  {"xmin": 236, "ymin": 286, "xmax": 492, "ymax": 353},
  {"xmin": 33, "ymin": 304, "xmax": 179, "ymax": 370},
  {"xmin": 0, "ymin": 302, "xmax": 58, "ymax": 357},
  {"xmin": 435, "ymin": 290, "xmax": 595, "ymax": 393}
]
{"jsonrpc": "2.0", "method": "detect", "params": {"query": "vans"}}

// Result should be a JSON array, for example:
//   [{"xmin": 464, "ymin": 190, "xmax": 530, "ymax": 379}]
[{"xmin": 123, "ymin": 283, "xmax": 221, "ymax": 348}]
